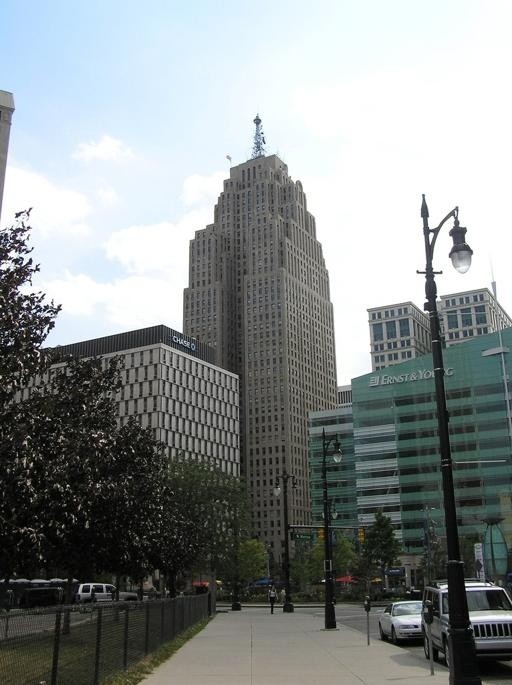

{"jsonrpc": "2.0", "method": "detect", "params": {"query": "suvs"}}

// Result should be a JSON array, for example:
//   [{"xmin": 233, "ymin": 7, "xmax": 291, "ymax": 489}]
[
  {"xmin": 74, "ymin": 582, "xmax": 138, "ymax": 602},
  {"xmin": 420, "ymin": 577, "xmax": 512, "ymax": 668}
]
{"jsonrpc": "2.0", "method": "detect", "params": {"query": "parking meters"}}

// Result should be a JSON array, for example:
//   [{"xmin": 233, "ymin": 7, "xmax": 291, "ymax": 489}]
[{"xmin": 363, "ymin": 595, "xmax": 371, "ymax": 646}]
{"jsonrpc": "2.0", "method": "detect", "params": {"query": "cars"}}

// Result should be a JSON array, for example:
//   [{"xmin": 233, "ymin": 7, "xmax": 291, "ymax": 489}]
[{"xmin": 378, "ymin": 599, "xmax": 439, "ymax": 645}]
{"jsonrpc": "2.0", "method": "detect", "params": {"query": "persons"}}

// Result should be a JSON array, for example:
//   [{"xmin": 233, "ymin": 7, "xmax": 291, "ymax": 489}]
[{"xmin": 266, "ymin": 582, "xmax": 278, "ymax": 615}]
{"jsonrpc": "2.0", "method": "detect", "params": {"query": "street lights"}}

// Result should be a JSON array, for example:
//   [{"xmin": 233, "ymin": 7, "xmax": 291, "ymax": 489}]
[
  {"xmin": 223, "ymin": 471, "xmax": 242, "ymax": 611},
  {"xmin": 317, "ymin": 425, "xmax": 343, "ymax": 630},
  {"xmin": 272, "ymin": 467, "xmax": 299, "ymax": 613},
  {"xmin": 415, "ymin": 192, "xmax": 482, "ymax": 685}
]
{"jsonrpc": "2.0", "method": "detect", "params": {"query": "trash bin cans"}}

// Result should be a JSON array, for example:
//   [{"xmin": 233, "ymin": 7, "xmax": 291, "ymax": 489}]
[{"xmin": 411, "ymin": 591, "xmax": 420, "ymax": 600}]
